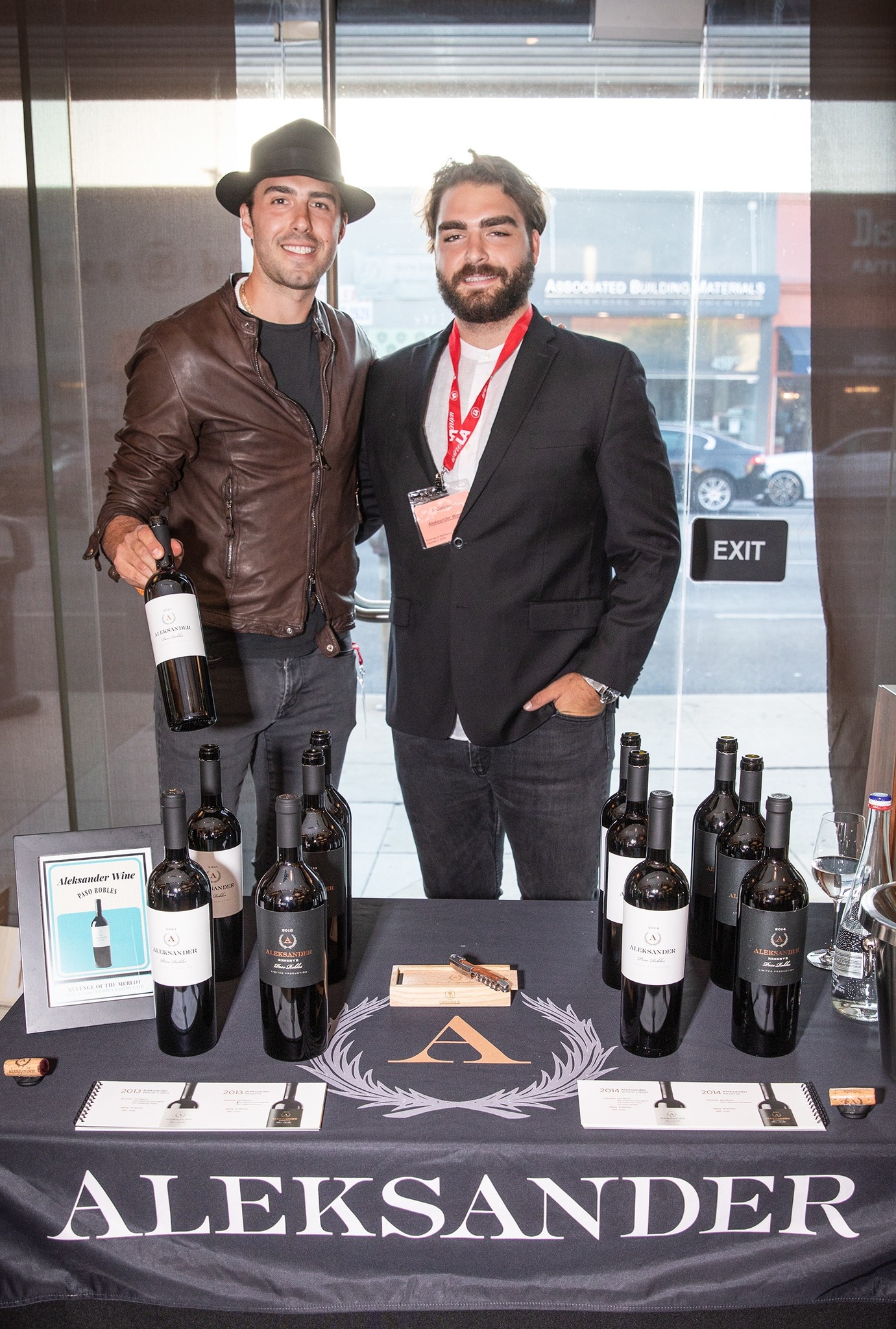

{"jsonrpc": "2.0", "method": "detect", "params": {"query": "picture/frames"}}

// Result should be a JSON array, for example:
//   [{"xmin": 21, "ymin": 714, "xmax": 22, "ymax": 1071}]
[{"xmin": 10, "ymin": 825, "xmax": 160, "ymax": 1037}]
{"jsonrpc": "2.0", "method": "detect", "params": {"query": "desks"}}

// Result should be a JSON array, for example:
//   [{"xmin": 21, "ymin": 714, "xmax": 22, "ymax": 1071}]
[{"xmin": 1, "ymin": 891, "xmax": 896, "ymax": 1328}]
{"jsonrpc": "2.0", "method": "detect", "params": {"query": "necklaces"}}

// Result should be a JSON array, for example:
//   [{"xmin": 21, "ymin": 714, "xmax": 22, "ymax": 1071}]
[{"xmin": 240, "ymin": 281, "xmax": 253, "ymax": 314}]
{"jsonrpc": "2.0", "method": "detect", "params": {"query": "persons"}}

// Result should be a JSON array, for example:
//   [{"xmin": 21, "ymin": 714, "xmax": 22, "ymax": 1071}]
[
  {"xmin": 355, "ymin": 152, "xmax": 680, "ymax": 903},
  {"xmin": 79, "ymin": 117, "xmax": 381, "ymax": 884}
]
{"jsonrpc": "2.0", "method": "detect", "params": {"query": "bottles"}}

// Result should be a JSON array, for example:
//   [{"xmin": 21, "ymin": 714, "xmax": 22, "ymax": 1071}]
[
  {"xmin": 146, "ymin": 745, "xmax": 245, "ymax": 1057},
  {"xmin": 597, "ymin": 732, "xmax": 689, "ymax": 1058},
  {"xmin": 254, "ymin": 729, "xmax": 353, "ymax": 1062},
  {"xmin": 688, "ymin": 736, "xmax": 810, "ymax": 1057},
  {"xmin": 831, "ymin": 791, "xmax": 893, "ymax": 1022},
  {"xmin": 143, "ymin": 515, "xmax": 217, "ymax": 718}
]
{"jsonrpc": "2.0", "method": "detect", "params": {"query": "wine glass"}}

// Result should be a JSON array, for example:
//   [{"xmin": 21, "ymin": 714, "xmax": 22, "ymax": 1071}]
[{"xmin": 806, "ymin": 811, "xmax": 867, "ymax": 970}]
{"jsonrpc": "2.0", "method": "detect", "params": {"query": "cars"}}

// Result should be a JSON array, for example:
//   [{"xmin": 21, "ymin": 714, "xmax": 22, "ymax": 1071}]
[
  {"xmin": 754, "ymin": 426, "xmax": 896, "ymax": 507},
  {"xmin": 655, "ymin": 425, "xmax": 766, "ymax": 514}
]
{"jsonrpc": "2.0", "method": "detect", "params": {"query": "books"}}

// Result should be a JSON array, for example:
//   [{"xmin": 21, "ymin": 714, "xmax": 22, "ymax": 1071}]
[
  {"xmin": 73, "ymin": 1080, "xmax": 328, "ymax": 1131},
  {"xmin": 576, "ymin": 1080, "xmax": 829, "ymax": 1132}
]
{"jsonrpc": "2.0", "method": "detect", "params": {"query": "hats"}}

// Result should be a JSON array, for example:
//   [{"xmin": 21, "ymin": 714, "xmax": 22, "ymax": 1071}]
[{"xmin": 214, "ymin": 117, "xmax": 376, "ymax": 225}]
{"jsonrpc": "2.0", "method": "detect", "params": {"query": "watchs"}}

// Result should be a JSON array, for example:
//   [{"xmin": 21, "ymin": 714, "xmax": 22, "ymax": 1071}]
[{"xmin": 582, "ymin": 675, "xmax": 622, "ymax": 704}]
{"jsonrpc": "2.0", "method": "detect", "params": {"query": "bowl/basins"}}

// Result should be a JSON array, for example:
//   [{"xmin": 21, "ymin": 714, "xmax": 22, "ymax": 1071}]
[{"xmin": 858, "ymin": 881, "xmax": 895, "ymax": 1082}]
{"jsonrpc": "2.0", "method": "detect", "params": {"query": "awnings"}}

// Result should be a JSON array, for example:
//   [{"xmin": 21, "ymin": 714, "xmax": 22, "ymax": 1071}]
[{"xmin": 778, "ymin": 327, "xmax": 896, "ymax": 377}]
